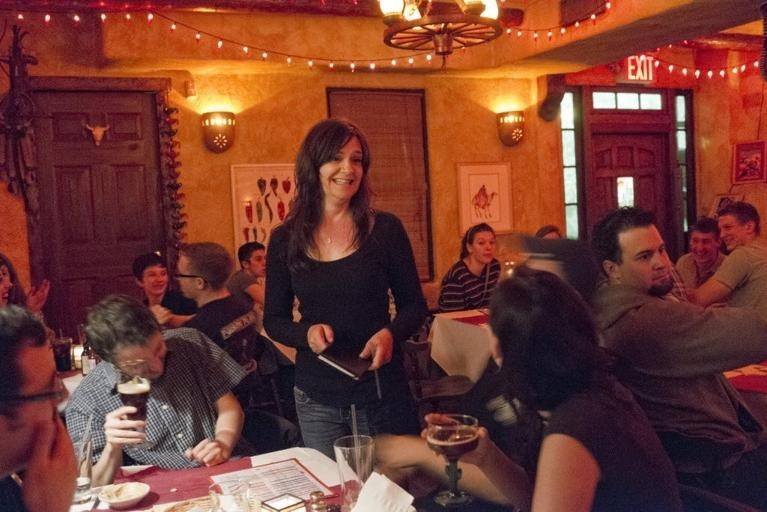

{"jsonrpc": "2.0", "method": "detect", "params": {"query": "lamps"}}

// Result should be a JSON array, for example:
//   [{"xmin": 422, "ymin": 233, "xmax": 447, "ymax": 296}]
[
  {"xmin": 200, "ymin": 112, "xmax": 236, "ymax": 154},
  {"xmin": 496, "ymin": 111, "xmax": 525, "ymax": 147},
  {"xmin": 377, "ymin": 0, "xmax": 503, "ymax": 73}
]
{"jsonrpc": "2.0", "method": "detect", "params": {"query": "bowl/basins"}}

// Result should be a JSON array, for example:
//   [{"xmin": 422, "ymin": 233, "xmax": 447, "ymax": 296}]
[{"xmin": 98, "ymin": 481, "xmax": 151, "ymax": 509}]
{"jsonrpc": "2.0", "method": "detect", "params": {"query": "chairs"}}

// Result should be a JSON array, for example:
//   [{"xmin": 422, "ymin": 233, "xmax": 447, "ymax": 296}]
[
  {"xmin": 244, "ymin": 339, "xmax": 284, "ymax": 417},
  {"xmin": 406, "ymin": 335, "xmax": 474, "ymax": 430}
]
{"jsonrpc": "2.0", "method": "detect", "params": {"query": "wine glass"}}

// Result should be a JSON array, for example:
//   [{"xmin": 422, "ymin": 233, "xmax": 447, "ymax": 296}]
[
  {"xmin": 427, "ymin": 414, "xmax": 479, "ymax": 508},
  {"xmin": 116, "ymin": 382, "xmax": 154, "ymax": 450}
]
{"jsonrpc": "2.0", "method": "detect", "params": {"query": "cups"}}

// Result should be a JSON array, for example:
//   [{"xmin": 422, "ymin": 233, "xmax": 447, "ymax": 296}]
[
  {"xmin": 332, "ymin": 435, "xmax": 374, "ymax": 512},
  {"xmin": 208, "ymin": 481, "xmax": 250, "ymax": 512},
  {"xmin": 70, "ymin": 440, "xmax": 92, "ymax": 505},
  {"xmin": 52, "ymin": 337, "xmax": 73, "ymax": 376}
]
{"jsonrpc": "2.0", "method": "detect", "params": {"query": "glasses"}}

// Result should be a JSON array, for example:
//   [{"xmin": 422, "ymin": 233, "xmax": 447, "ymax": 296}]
[
  {"xmin": 171, "ymin": 271, "xmax": 199, "ymax": 282},
  {"xmin": 0, "ymin": 372, "xmax": 69, "ymax": 407}
]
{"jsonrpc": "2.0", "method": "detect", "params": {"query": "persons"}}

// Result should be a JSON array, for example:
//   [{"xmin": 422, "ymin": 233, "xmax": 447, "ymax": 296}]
[
  {"xmin": 1, "ymin": 242, "xmax": 302, "ymax": 511},
  {"xmin": 376, "ymin": 201, "xmax": 766, "ymax": 511},
  {"xmin": 263, "ymin": 120, "xmax": 428, "ymax": 461}
]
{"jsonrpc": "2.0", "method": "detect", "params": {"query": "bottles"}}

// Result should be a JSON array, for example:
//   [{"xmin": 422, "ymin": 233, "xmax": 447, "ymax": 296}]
[
  {"xmin": 81, "ymin": 346, "xmax": 101, "ymax": 377},
  {"xmin": 307, "ymin": 490, "xmax": 332, "ymax": 512}
]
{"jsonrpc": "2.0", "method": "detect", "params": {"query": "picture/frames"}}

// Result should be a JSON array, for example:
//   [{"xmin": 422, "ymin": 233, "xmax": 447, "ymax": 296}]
[
  {"xmin": 732, "ymin": 140, "xmax": 767, "ymax": 185},
  {"xmin": 707, "ymin": 192, "xmax": 745, "ymax": 221},
  {"xmin": 231, "ymin": 163, "xmax": 299, "ymax": 272},
  {"xmin": 455, "ymin": 160, "xmax": 514, "ymax": 237}
]
